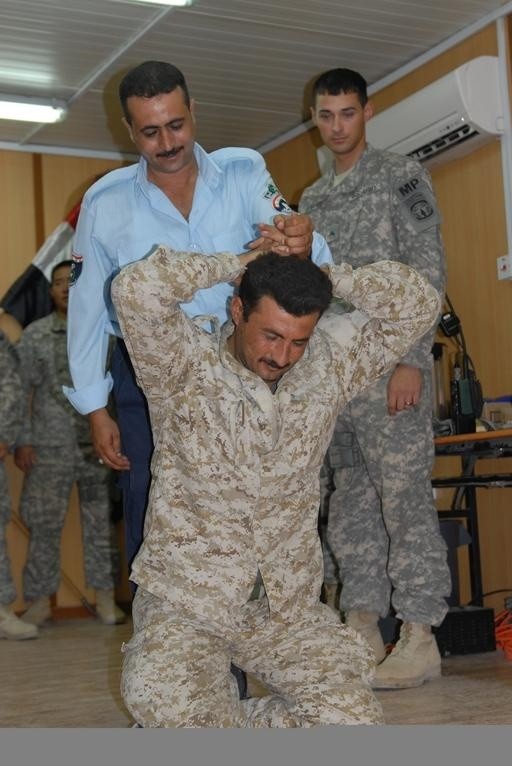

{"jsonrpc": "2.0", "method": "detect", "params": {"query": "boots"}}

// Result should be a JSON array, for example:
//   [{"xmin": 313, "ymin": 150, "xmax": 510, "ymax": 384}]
[
  {"xmin": 0, "ymin": 604, "xmax": 39, "ymax": 641},
  {"xmin": 343, "ymin": 610, "xmax": 386, "ymax": 667},
  {"xmin": 19, "ymin": 596, "xmax": 53, "ymax": 627},
  {"xmin": 370, "ymin": 621, "xmax": 442, "ymax": 688},
  {"xmin": 324, "ymin": 582, "xmax": 346, "ymax": 626},
  {"xmin": 96, "ymin": 588, "xmax": 126, "ymax": 625}
]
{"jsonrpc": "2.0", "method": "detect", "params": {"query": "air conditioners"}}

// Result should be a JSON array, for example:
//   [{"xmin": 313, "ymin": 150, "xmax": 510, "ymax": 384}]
[{"xmin": 314, "ymin": 52, "xmax": 502, "ymax": 189}]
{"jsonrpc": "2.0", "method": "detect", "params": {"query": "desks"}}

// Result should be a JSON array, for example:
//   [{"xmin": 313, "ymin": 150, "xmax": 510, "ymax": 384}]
[{"xmin": 433, "ymin": 427, "xmax": 512, "ymax": 608}]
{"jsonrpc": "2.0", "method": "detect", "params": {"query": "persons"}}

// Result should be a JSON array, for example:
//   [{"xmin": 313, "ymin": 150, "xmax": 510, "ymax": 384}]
[
  {"xmin": 10, "ymin": 256, "xmax": 128, "ymax": 631},
  {"xmin": 290, "ymin": 64, "xmax": 455, "ymax": 690},
  {"xmin": 57, "ymin": 56, "xmax": 337, "ymax": 611},
  {"xmin": 106, "ymin": 209, "xmax": 445, "ymax": 732},
  {"xmin": 0, "ymin": 325, "xmax": 42, "ymax": 644}
]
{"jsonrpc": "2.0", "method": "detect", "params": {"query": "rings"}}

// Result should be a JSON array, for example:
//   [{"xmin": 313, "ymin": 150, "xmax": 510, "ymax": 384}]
[
  {"xmin": 404, "ymin": 400, "xmax": 413, "ymax": 406},
  {"xmin": 97, "ymin": 457, "xmax": 104, "ymax": 465}
]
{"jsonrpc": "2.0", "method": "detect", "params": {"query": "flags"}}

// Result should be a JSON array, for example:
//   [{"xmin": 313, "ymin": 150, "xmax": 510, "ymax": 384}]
[{"xmin": 0, "ymin": 197, "xmax": 83, "ymax": 330}]
